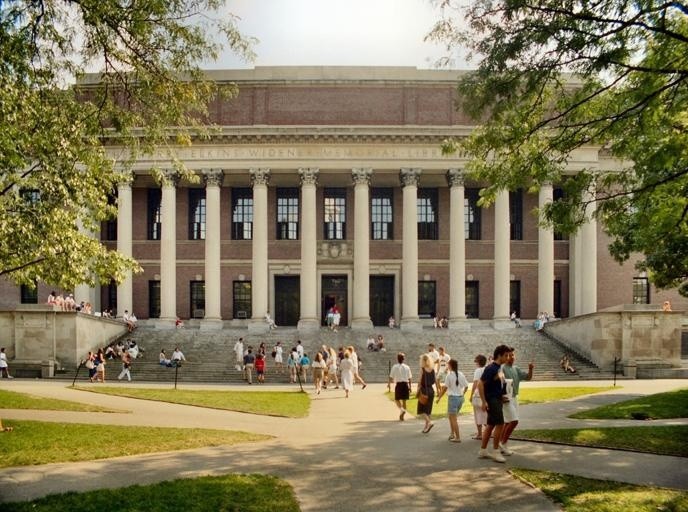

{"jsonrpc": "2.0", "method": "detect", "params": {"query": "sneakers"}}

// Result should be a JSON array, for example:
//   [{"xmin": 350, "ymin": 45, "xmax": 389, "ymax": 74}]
[
  {"xmin": 476, "ymin": 450, "xmax": 491, "ymax": 459},
  {"xmin": 235, "ymin": 364, "xmax": 264, "ymax": 385},
  {"xmin": 90, "ymin": 377, "xmax": 106, "ymax": 383},
  {"xmin": 399, "ymin": 409, "xmax": 406, "ymax": 422},
  {"xmin": 499, "ymin": 441, "xmax": 515, "ymax": 456},
  {"xmin": 447, "ymin": 434, "xmax": 461, "ymax": 443},
  {"xmin": 491, "ymin": 448, "xmax": 507, "ymax": 463},
  {"xmin": 472, "ymin": 435, "xmax": 483, "ymax": 440},
  {"xmin": 314, "ymin": 384, "xmax": 367, "ymax": 398},
  {"xmin": 7, "ymin": 375, "xmax": 14, "ymax": 379},
  {"xmin": 421, "ymin": 422, "xmax": 435, "ymax": 434}
]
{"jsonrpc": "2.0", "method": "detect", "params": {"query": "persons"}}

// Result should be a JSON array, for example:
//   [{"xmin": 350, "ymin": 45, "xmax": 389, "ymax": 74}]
[
  {"xmin": 159, "ymin": 347, "xmax": 186, "ymax": 367},
  {"xmin": 325, "ymin": 304, "xmax": 341, "ymax": 334},
  {"xmin": 510, "ymin": 310, "xmax": 522, "ymax": 328},
  {"xmin": 233, "ymin": 337, "xmax": 267, "ymax": 385},
  {"xmin": 86, "ymin": 338, "xmax": 139, "ymax": 383},
  {"xmin": 389, "ymin": 343, "xmax": 535, "ymax": 463},
  {"xmin": 287, "ymin": 340, "xmax": 310, "ymax": 384},
  {"xmin": 47, "ymin": 290, "xmax": 138, "ymax": 333},
  {"xmin": 560, "ymin": 356, "xmax": 576, "ymax": 374},
  {"xmin": 264, "ymin": 311, "xmax": 277, "ymax": 330},
  {"xmin": 533, "ymin": 311, "xmax": 549, "ymax": 330},
  {"xmin": 433, "ymin": 313, "xmax": 448, "ymax": 329},
  {"xmin": 272, "ymin": 341, "xmax": 285, "ymax": 375},
  {"xmin": 367, "ymin": 334, "xmax": 384, "ymax": 352},
  {"xmin": 176, "ymin": 316, "xmax": 184, "ymax": 329},
  {"xmin": 0, "ymin": 348, "xmax": 14, "ymax": 378},
  {"xmin": 312, "ymin": 344, "xmax": 367, "ymax": 398},
  {"xmin": 388, "ymin": 317, "xmax": 394, "ymax": 329}
]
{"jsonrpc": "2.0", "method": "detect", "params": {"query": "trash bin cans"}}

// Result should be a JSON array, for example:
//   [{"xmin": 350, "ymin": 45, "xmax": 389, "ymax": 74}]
[
  {"xmin": 40, "ymin": 359, "xmax": 54, "ymax": 378},
  {"xmin": 623, "ymin": 365, "xmax": 637, "ymax": 379}
]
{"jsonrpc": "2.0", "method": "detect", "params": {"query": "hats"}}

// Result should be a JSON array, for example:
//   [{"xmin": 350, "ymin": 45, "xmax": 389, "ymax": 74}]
[{"xmin": 69, "ymin": 294, "xmax": 74, "ymax": 297}]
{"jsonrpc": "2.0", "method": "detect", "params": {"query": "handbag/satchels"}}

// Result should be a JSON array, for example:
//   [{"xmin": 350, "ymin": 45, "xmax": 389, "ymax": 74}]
[
  {"xmin": 418, "ymin": 389, "xmax": 429, "ymax": 405},
  {"xmin": 272, "ymin": 346, "xmax": 277, "ymax": 358}
]
